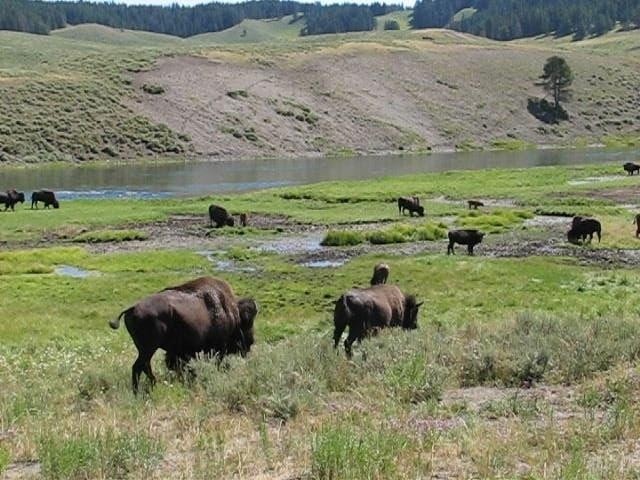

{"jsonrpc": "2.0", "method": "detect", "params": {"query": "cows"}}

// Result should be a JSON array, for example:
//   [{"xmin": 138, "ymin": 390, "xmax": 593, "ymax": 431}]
[
  {"xmin": 31, "ymin": 190, "xmax": 59, "ymax": 209},
  {"xmin": 0, "ymin": 188, "xmax": 25, "ymax": 212},
  {"xmin": 108, "ymin": 275, "xmax": 258, "ymax": 398},
  {"xmin": 567, "ymin": 216, "xmax": 602, "ymax": 245},
  {"xmin": 332, "ymin": 263, "xmax": 425, "ymax": 360},
  {"xmin": 631, "ymin": 213, "xmax": 640, "ymax": 237},
  {"xmin": 208, "ymin": 204, "xmax": 249, "ymax": 229},
  {"xmin": 623, "ymin": 162, "xmax": 640, "ymax": 176},
  {"xmin": 447, "ymin": 229, "xmax": 485, "ymax": 256},
  {"xmin": 396, "ymin": 195, "xmax": 425, "ymax": 218},
  {"xmin": 468, "ymin": 200, "xmax": 484, "ymax": 210}
]
